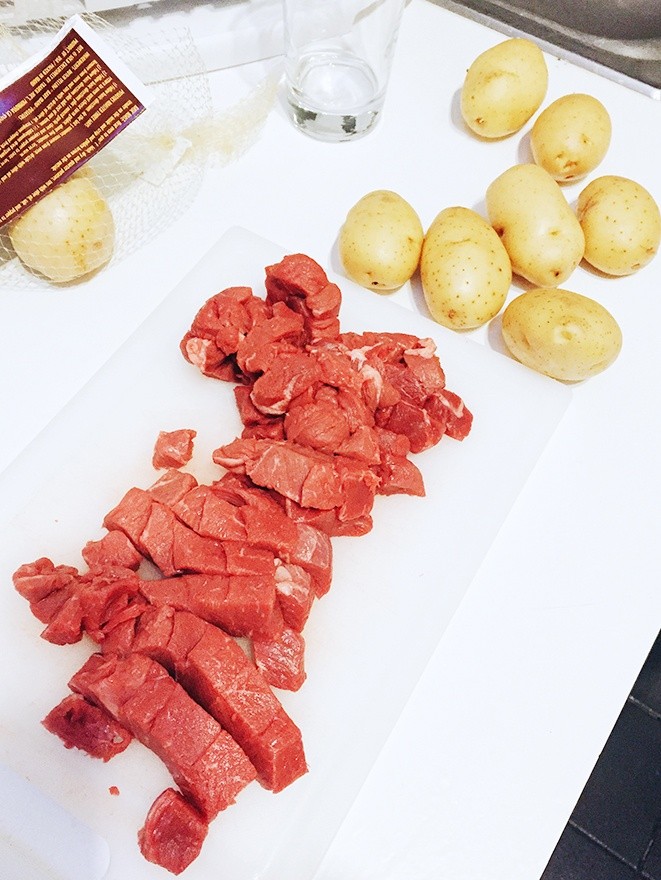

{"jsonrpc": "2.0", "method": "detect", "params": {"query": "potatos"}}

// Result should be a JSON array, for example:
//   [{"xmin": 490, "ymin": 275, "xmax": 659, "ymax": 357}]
[
  {"xmin": 338, "ymin": 37, "xmax": 660, "ymax": 380},
  {"xmin": 6, "ymin": 178, "xmax": 114, "ymax": 283}
]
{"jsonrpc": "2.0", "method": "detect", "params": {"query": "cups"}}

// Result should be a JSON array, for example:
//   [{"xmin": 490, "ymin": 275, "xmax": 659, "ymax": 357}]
[{"xmin": 280, "ymin": 0, "xmax": 407, "ymax": 144}]
